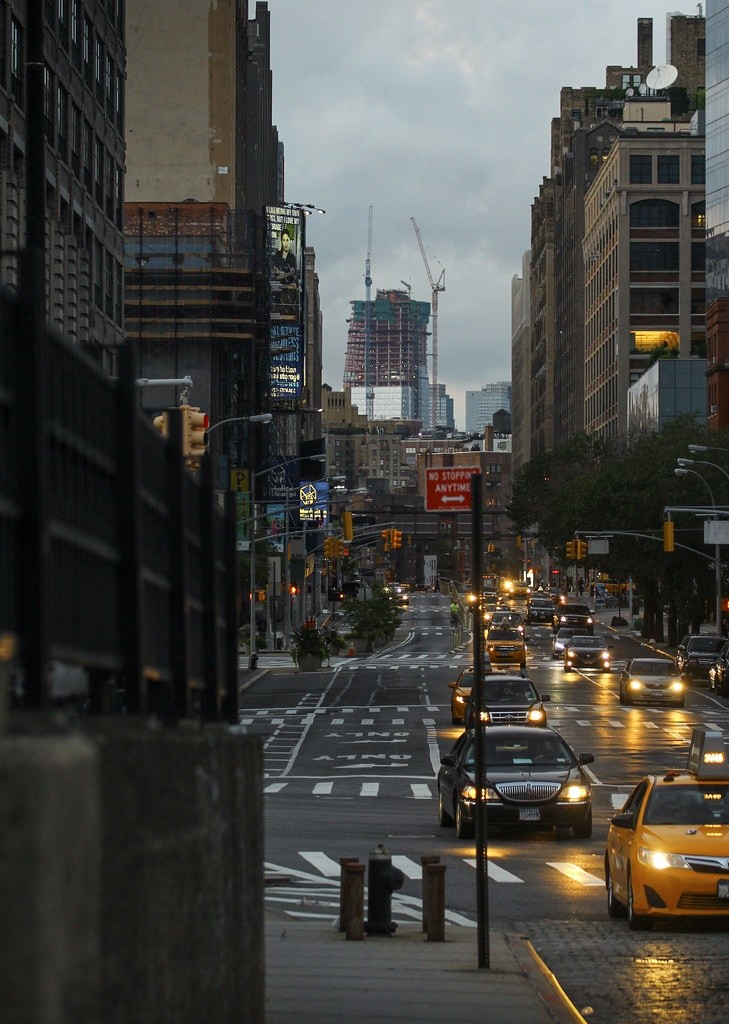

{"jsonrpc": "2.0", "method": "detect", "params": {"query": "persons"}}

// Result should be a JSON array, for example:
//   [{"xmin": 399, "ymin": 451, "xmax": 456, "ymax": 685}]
[
  {"xmin": 578, "ymin": 576, "xmax": 584, "ymax": 596},
  {"xmin": 434, "ymin": 578, "xmax": 440, "ymax": 592},
  {"xmin": 450, "ymin": 599, "xmax": 460, "ymax": 622}
]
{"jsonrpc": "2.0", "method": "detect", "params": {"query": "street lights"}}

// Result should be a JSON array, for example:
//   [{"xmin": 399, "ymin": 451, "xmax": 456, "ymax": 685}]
[
  {"xmin": 250, "ymin": 454, "xmax": 329, "ymax": 669},
  {"xmin": 674, "ymin": 467, "xmax": 723, "ymax": 636},
  {"xmin": 282, "ymin": 475, "xmax": 373, "ymax": 651}
]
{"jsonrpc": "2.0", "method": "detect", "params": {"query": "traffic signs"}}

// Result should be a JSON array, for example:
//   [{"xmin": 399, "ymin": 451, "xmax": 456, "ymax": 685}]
[{"xmin": 424, "ymin": 465, "xmax": 482, "ymax": 513}]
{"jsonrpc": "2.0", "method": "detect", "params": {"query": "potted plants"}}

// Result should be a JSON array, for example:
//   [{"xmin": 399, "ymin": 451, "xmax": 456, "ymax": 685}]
[
  {"xmin": 289, "ymin": 627, "xmax": 330, "ymax": 671},
  {"xmin": 344, "ymin": 583, "xmax": 407, "ymax": 653}
]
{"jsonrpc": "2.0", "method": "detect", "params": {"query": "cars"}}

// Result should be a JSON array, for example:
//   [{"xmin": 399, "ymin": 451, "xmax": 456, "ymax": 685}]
[
  {"xmin": 464, "ymin": 674, "xmax": 551, "ymax": 732},
  {"xmin": 381, "ymin": 581, "xmax": 434, "ymax": 606},
  {"xmin": 618, "ymin": 657, "xmax": 688, "ymax": 708},
  {"xmin": 604, "ymin": 725, "xmax": 729, "ymax": 931},
  {"xmin": 459, "ymin": 573, "xmax": 596, "ymax": 669},
  {"xmin": 447, "ymin": 664, "xmax": 522, "ymax": 726},
  {"xmin": 708, "ymin": 640, "xmax": 729, "ymax": 699},
  {"xmin": 674, "ymin": 632, "xmax": 728, "ymax": 679},
  {"xmin": 563, "ymin": 635, "xmax": 614, "ymax": 673},
  {"xmin": 434, "ymin": 723, "xmax": 597, "ymax": 840},
  {"xmin": 548, "ymin": 627, "xmax": 592, "ymax": 659}
]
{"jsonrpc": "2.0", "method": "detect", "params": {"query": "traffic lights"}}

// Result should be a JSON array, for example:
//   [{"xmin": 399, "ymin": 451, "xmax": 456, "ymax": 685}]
[
  {"xmin": 393, "ymin": 530, "xmax": 403, "ymax": 548},
  {"xmin": 577, "ymin": 540, "xmax": 587, "ymax": 560},
  {"xmin": 566, "ymin": 539, "xmax": 576, "ymax": 560},
  {"xmin": 323, "ymin": 537, "xmax": 333, "ymax": 558},
  {"xmin": 289, "ymin": 584, "xmax": 297, "ymax": 595},
  {"xmin": 178, "ymin": 404, "xmax": 209, "ymax": 460},
  {"xmin": 335, "ymin": 539, "xmax": 344, "ymax": 557},
  {"xmin": 487, "ymin": 543, "xmax": 495, "ymax": 553},
  {"xmin": 382, "ymin": 530, "xmax": 389, "ymax": 539},
  {"xmin": 255, "ymin": 590, "xmax": 267, "ymax": 602}
]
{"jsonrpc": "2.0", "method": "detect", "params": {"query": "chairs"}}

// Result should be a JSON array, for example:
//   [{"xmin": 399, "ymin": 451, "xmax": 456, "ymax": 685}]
[{"xmin": 483, "ymin": 740, "xmax": 497, "ymax": 757}]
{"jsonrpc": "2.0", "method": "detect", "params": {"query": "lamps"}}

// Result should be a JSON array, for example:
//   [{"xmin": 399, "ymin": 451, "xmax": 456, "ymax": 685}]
[{"xmin": 283, "ymin": 201, "xmax": 326, "ymax": 216}]
